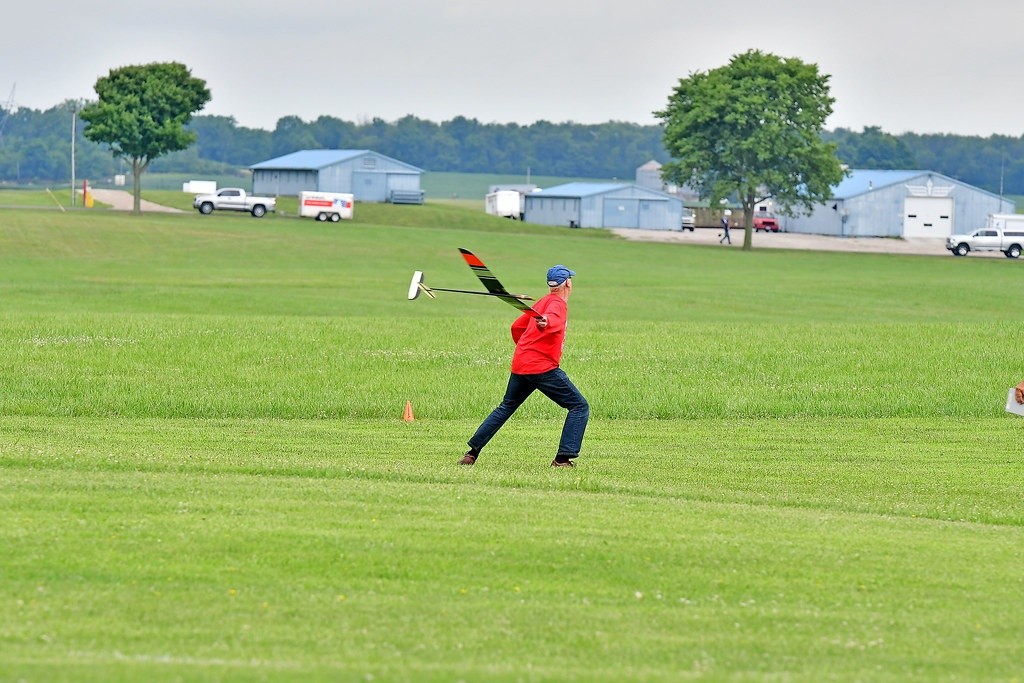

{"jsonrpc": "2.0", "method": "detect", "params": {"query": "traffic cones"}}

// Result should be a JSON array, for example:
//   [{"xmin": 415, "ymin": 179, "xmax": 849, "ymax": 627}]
[{"xmin": 404, "ymin": 401, "xmax": 414, "ymax": 421}]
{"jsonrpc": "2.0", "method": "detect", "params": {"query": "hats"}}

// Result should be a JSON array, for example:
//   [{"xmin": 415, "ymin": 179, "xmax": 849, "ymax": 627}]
[{"xmin": 547, "ymin": 265, "xmax": 576, "ymax": 288}]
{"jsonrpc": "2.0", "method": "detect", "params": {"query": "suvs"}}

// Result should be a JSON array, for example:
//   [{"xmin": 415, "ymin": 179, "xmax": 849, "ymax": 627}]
[
  {"xmin": 752, "ymin": 211, "xmax": 779, "ymax": 233},
  {"xmin": 683, "ymin": 208, "xmax": 696, "ymax": 231}
]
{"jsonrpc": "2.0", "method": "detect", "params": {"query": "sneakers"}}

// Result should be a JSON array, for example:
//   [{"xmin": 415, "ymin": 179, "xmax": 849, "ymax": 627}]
[
  {"xmin": 551, "ymin": 460, "xmax": 574, "ymax": 468},
  {"xmin": 459, "ymin": 454, "xmax": 475, "ymax": 466}
]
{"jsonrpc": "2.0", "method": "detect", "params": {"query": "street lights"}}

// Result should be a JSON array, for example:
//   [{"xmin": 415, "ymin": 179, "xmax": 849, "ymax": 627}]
[
  {"xmin": 67, "ymin": 107, "xmax": 76, "ymax": 205},
  {"xmin": 1000, "ymin": 144, "xmax": 1006, "ymax": 215}
]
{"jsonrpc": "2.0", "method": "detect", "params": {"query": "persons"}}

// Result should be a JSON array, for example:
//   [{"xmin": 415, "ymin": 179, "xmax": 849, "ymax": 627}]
[
  {"xmin": 720, "ymin": 218, "xmax": 732, "ymax": 245},
  {"xmin": 457, "ymin": 265, "xmax": 590, "ymax": 467}
]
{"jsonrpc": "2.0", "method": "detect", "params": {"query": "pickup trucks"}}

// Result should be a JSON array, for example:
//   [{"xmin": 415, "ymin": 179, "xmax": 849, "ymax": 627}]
[
  {"xmin": 945, "ymin": 228, "xmax": 1024, "ymax": 258},
  {"xmin": 194, "ymin": 187, "xmax": 276, "ymax": 218}
]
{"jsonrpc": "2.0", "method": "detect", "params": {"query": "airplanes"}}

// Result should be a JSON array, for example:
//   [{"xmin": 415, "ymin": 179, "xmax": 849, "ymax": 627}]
[{"xmin": 406, "ymin": 248, "xmax": 548, "ymax": 323}]
